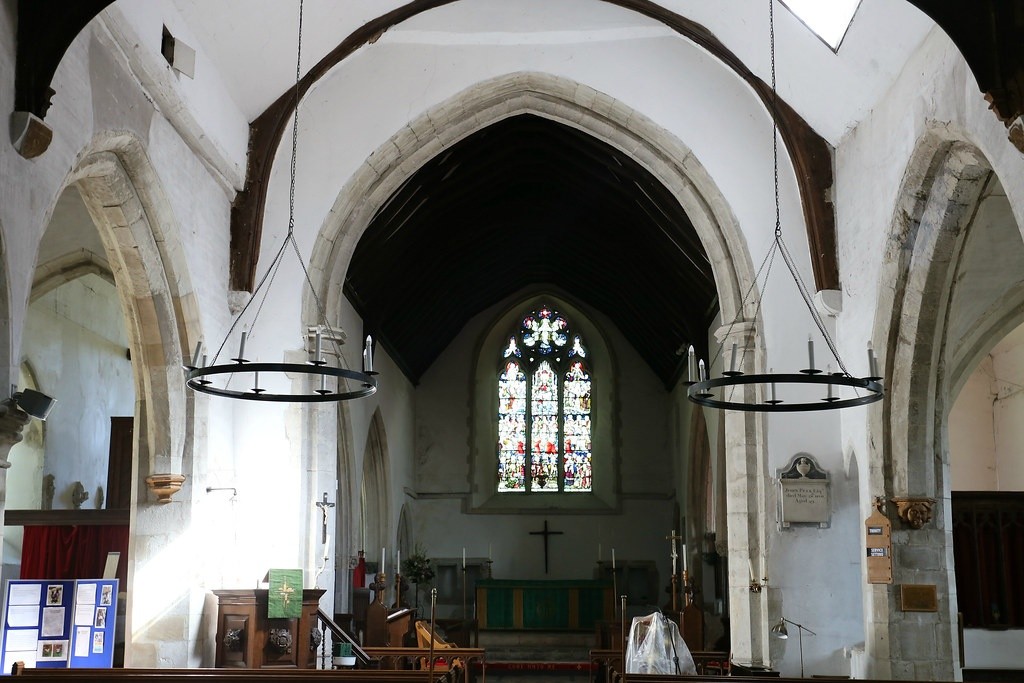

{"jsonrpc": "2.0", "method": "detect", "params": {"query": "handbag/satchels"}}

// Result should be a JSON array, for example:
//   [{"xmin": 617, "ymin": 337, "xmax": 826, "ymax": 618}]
[{"xmin": 625, "ymin": 613, "xmax": 698, "ymax": 675}]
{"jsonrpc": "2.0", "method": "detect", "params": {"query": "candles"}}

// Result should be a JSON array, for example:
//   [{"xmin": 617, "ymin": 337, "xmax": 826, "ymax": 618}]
[
  {"xmin": 397, "ymin": 550, "xmax": 400, "ymax": 574},
  {"xmin": 598, "ymin": 544, "xmax": 601, "ymax": 560},
  {"xmin": 382, "ymin": 548, "xmax": 385, "ymax": 574},
  {"xmin": 612, "ymin": 548, "xmax": 615, "ymax": 568},
  {"xmin": 462, "ymin": 547, "xmax": 465, "ymax": 568},
  {"xmin": 748, "ymin": 558, "xmax": 754, "ymax": 581},
  {"xmin": 672, "ymin": 554, "xmax": 676, "ymax": 575},
  {"xmin": 488, "ymin": 542, "xmax": 491, "ymax": 561},
  {"xmin": 683, "ymin": 544, "xmax": 687, "ymax": 571}
]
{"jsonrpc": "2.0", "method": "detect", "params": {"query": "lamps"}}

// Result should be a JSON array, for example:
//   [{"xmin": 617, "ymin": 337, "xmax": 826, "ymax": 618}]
[
  {"xmin": 11, "ymin": 389, "xmax": 58, "ymax": 421},
  {"xmin": 770, "ymin": 618, "xmax": 816, "ymax": 678},
  {"xmin": 685, "ymin": 0, "xmax": 884, "ymax": 414},
  {"xmin": 182, "ymin": 0, "xmax": 380, "ymax": 404}
]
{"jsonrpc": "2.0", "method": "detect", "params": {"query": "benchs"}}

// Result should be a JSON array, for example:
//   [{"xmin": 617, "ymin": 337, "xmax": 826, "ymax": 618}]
[
  {"xmin": 588, "ymin": 650, "xmax": 852, "ymax": 683},
  {"xmin": 0, "ymin": 647, "xmax": 488, "ymax": 683}
]
